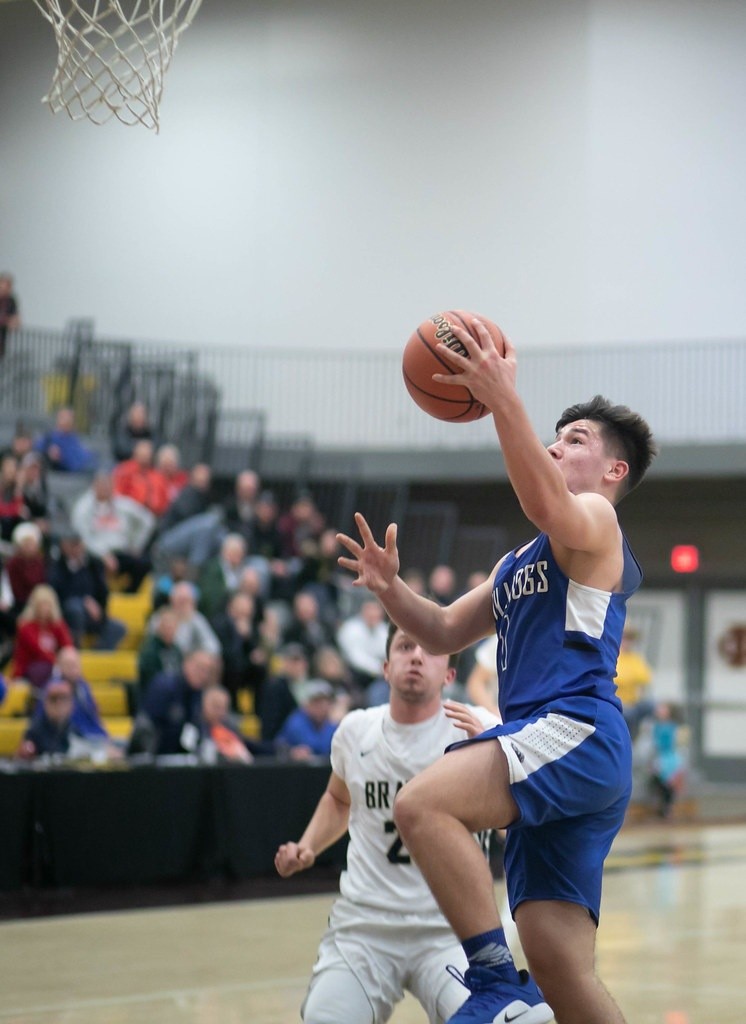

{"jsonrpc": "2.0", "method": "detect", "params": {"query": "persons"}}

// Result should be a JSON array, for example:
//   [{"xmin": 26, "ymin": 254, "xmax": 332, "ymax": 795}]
[
  {"xmin": 275, "ymin": 593, "xmax": 507, "ymax": 1024},
  {"xmin": 0, "ymin": 269, "xmax": 688, "ymax": 819},
  {"xmin": 335, "ymin": 318, "xmax": 658, "ymax": 1024}
]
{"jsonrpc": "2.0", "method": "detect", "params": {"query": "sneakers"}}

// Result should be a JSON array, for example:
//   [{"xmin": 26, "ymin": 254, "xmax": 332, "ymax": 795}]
[{"xmin": 443, "ymin": 964, "xmax": 554, "ymax": 1024}]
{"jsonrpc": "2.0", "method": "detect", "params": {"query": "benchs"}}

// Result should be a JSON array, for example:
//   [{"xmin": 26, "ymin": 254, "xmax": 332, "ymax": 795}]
[{"xmin": 0, "ymin": 591, "xmax": 155, "ymax": 758}]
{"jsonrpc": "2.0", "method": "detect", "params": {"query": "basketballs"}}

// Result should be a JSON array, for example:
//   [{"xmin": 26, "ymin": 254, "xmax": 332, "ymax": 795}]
[{"xmin": 401, "ymin": 307, "xmax": 509, "ymax": 426}]
